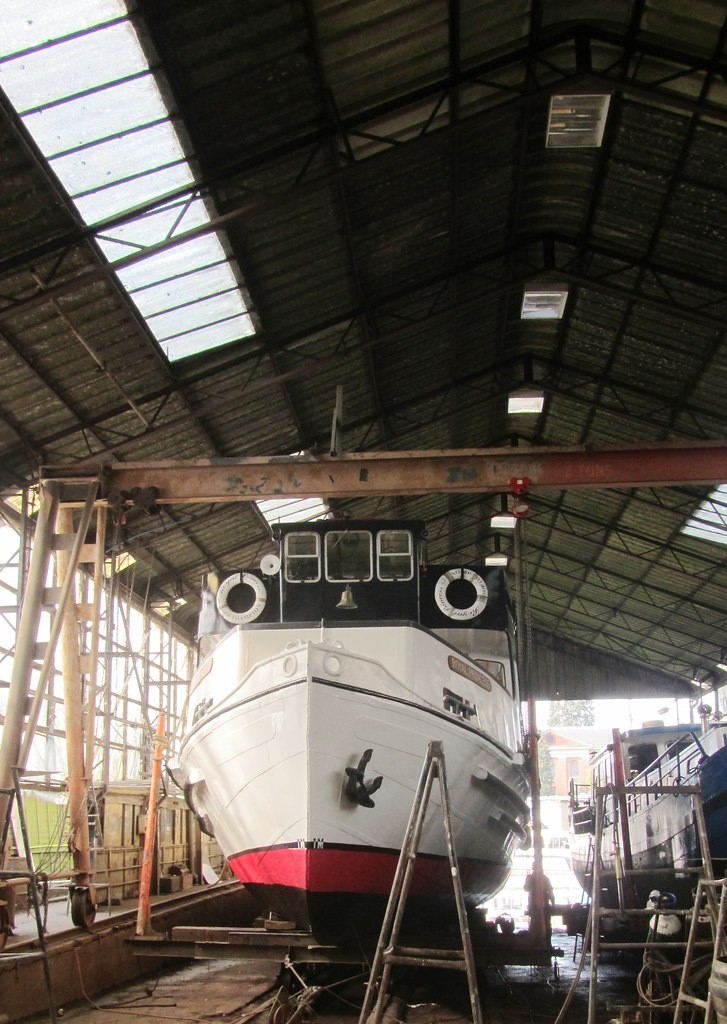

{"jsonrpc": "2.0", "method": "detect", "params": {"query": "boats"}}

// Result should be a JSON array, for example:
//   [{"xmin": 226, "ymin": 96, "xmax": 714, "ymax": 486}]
[
  {"xmin": 567, "ymin": 720, "xmax": 727, "ymax": 908},
  {"xmin": 167, "ymin": 515, "xmax": 533, "ymax": 946}
]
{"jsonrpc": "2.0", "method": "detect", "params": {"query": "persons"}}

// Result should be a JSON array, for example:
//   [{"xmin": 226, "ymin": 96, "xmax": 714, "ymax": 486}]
[{"xmin": 524, "ymin": 862, "xmax": 555, "ymax": 935}]
